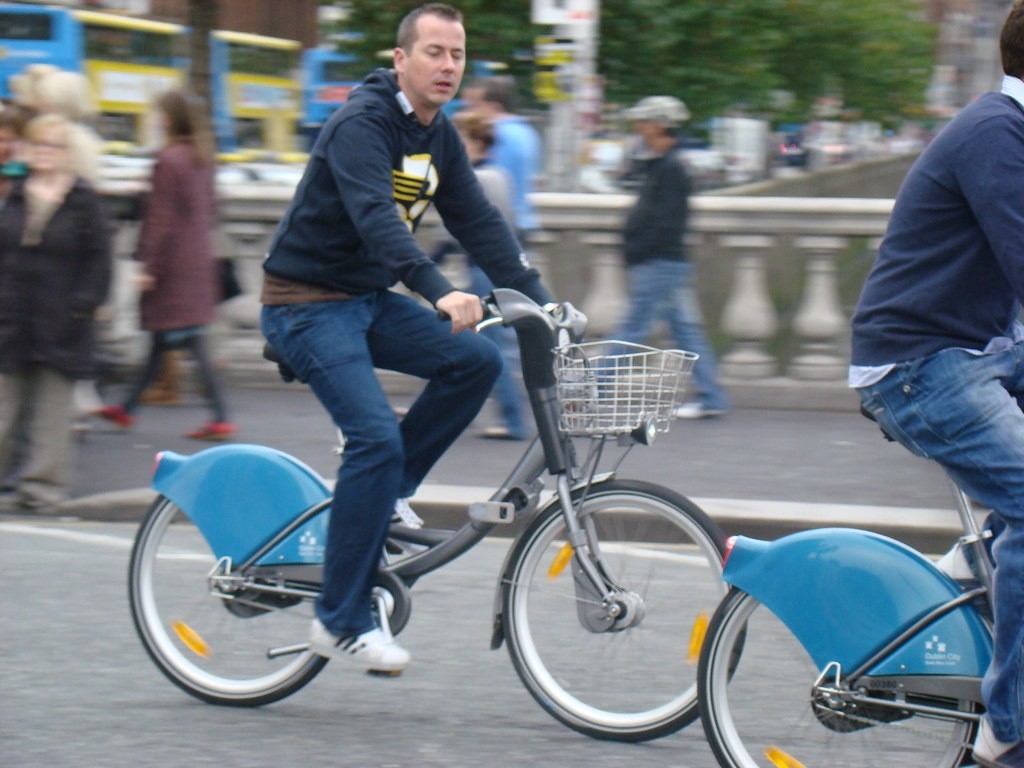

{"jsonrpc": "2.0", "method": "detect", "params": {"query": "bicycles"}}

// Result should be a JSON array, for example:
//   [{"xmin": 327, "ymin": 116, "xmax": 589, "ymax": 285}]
[
  {"xmin": 128, "ymin": 290, "xmax": 751, "ymax": 739},
  {"xmin": 695, "ymin": 398, "xmax": 995, "ymax": 768}
]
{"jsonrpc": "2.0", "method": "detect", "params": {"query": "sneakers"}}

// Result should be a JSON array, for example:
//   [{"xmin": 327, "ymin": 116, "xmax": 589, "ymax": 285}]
[
  {"xmin": 971, "ymin": 712, "xmax": 1024, "ymax": 768},
  {"xmin": 387, "ymin": 498, "xmax": 430, "ymax": 558},
  {"xmin": 308, "ymin": 617, "xmax": 411, "ymax": 673},
  {"xmin": 934, "ymin": 538, "xmax": 986, "ymax": 590}
]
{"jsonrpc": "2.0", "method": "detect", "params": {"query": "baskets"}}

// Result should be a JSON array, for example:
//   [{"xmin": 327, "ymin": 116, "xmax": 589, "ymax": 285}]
[{"xmin": 550, "ymin": 340, "xmax": 699, "ymax": 437}]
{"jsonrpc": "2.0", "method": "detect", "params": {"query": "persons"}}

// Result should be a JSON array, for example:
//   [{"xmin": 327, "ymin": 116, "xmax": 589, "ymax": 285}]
[
  {"xmin": 845, "ymin": 0, "xmax": 1024, "ymax": 768},
  {"xmin": 449, "ymin": 73, "xmax": 542, "ymax": 441},
  {"xmin": 563, "ymin": 92, "xmax": 735, "ymax": 423},
  {"xmin": 86, "ymin": 88, "xmax": 245, "ymax": 442},
  {"xmin": 259, "ymin": 2, "xmax": 562, "ymax": 675},
  {"xmin": 0, "ymin": 69, "xmax": 116, "ymax": 510}
]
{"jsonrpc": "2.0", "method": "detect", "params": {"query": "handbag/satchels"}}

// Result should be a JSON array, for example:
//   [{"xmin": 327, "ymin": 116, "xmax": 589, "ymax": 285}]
[{"xmin": 211, "ymin": 250, "xmax": 244, "ymax": 305}]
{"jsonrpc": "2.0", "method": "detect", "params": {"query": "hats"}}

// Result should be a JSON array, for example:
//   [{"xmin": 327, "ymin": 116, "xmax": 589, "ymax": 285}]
[
  {"xmin": 28, "ymin": 62, "xmax": 84, "ymax": 108},
  {"xmin": 621, "ymin": 94, "xmax": 691, "ymax": 121}
]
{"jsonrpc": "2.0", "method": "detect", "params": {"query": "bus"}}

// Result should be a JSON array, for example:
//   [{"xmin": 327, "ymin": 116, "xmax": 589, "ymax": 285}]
[
  {"xmin": 0, "ymin": 4, "xmax": 301, "ymax": 153},
  {"xmin": 299, "ymin": 33, "xmax": 504, "ymax": 144}
]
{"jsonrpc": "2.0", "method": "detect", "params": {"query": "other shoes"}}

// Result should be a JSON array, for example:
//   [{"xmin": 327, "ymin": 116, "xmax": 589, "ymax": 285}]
[
  {"xmin": 182, "ymin": 421, "xmax": 239, "ymax": 440},
  {"xmin": 18, "ymin": 479, "xmax": 70, "ymax": 506},
  {"xmin": 87, "ymin": 404, "xmax": 140, "ymax": 428},
  {"xmin": 666, "ymin": 398, "xmax": 730, "ymax": 419},
  {"xmin": 476, "ymin": 422, "xmax": 524, "ymax": 440}
]
